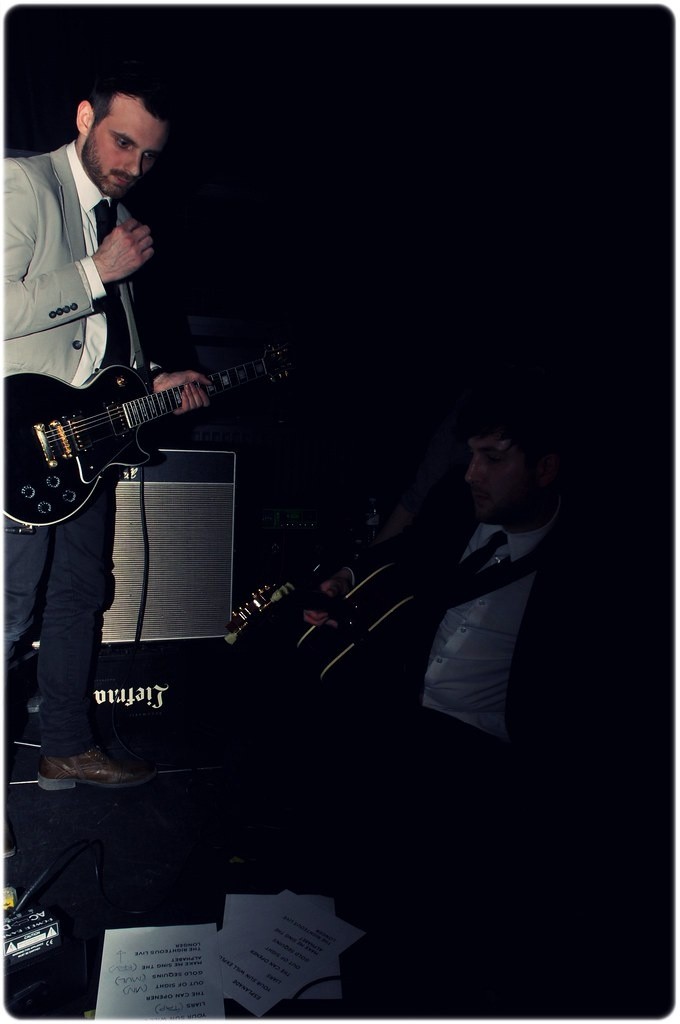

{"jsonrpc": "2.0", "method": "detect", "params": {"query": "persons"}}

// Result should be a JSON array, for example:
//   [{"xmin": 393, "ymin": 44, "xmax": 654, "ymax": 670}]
[
  {"xmin": 5, "ymin": 71, "xmax": 209, "ymax": 856},
  {"xmin": 303, "ymin": 349, "xmax": 614, "ymax": 797}
]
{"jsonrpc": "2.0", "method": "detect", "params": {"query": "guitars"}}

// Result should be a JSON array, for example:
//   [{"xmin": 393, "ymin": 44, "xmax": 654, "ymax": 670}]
[
  {"xmin": 223, "ymin": 559, "xmax": 418, "ymax": 687},
  {"xmin": 4, "ymin": 342, "xmax": 322, "ymax": 528}
]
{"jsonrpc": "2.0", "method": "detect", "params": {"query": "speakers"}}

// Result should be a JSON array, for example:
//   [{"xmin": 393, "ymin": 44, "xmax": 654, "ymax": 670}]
[{"xmin": 97, "ymin": 447, "xmax": 240, "ymax": 660}]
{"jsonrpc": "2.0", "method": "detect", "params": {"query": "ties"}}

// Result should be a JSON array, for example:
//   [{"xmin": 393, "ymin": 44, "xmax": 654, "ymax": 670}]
[
  {"xmin": 457, "ymin": 530, "xmax": 508, "ymax": 580},
  {"xmin": 93, "ymin": 197, "xmax": 131, "ymax": 370}
]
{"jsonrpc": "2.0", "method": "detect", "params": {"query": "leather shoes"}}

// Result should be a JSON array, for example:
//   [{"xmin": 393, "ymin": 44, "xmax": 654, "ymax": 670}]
[
  {"xmin": 37, "ymin": 744, "xmax": 158, "ymax": 790},
  {"xmin": 5, "ymin": 819, "xmax": 16, "ymax": 858}
]
